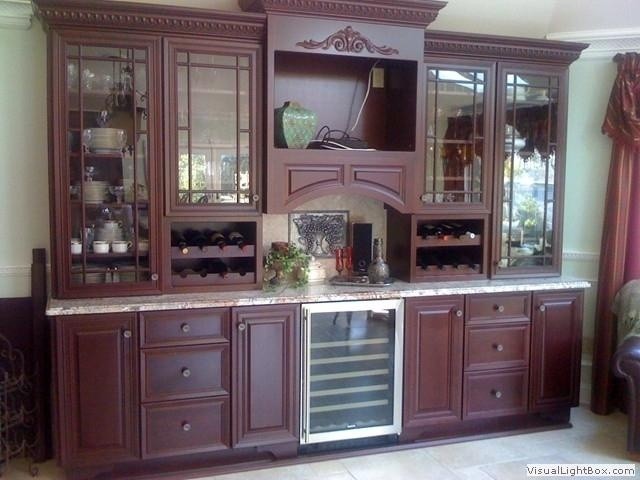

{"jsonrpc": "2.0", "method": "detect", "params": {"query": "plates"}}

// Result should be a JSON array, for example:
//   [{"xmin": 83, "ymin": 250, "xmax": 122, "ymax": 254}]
[{"xmin": 72, "ymin": 261, "xmax": 144, "ymax": 283}]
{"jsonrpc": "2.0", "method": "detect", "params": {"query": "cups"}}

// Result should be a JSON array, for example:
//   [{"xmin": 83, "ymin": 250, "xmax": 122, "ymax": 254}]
[
  {"xmin": 71, "ymin": 237, "xmax": 82, "ymax": 255},
  {"xmin": 104, "ymin": 219, "xmax": 123, "ymax": 229},
  {"xmin": 92, "ymin": 240, "xmax": 133, "ymax": 254}
]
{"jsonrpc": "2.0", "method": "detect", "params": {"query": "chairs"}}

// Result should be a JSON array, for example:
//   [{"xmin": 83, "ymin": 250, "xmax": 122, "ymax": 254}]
[{"xmin": 609, "ymin": 278, "xmax": 640, "ymax": 454}]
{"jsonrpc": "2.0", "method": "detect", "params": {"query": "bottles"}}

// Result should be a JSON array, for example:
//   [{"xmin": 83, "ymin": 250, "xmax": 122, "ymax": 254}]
[
  {"xmin": 367, "ymin": 238, "xmax": 390, "ymax": 284},
  {"xmin": 420, "ymin": 222, "xmax": 476, "ymax": 241},
  {"xmin": 171, "ymin": 228, "xmax": 249, "ymax": 255},
  {"xmin": 212, "ymin": 261, "xmax": 230, "ymax": 281}
]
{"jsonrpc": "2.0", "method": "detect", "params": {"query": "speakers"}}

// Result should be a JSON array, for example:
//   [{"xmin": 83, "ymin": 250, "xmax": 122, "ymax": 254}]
[{"xmin": 352, "ymin": 223, "xmax": 373, "ymax": 271}]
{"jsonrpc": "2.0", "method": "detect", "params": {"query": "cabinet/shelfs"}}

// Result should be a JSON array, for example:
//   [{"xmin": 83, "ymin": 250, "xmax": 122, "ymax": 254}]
[{"xmin": 30, "ymin": 1, "xmax": 593, "ymax": 479}]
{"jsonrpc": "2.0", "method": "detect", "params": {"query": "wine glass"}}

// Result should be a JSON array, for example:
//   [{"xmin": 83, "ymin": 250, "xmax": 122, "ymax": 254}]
[
  {"xmin": 85, "ymin": 165, "xmax": 95, "ymax": 185},
  {"xmin": 108, "ymin": 185, "xmax": 125, "ymax": 204},
  {"xmin": 79, "ymin": 226, "xmax": 95, "ymax": 254}
]
